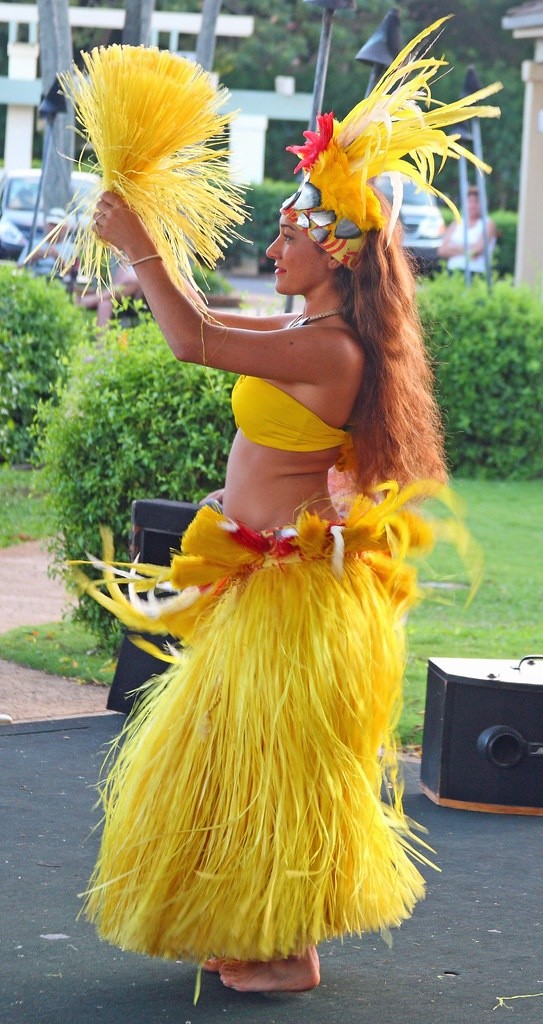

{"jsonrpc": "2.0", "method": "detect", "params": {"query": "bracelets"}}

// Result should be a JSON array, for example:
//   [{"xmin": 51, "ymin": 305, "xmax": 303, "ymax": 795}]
[{"xmin": 127, "ymin": 254, "xmax": 163, "ymax": 265}]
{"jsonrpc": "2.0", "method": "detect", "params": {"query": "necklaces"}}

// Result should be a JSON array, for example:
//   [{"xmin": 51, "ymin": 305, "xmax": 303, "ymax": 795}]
[{"xmin": 286, "ymin": 308, "xmax": 345, "ymax": 329}]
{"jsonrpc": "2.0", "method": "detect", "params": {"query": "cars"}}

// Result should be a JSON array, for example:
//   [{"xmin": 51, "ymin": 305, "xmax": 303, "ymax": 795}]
[
  {"xmin": 368, "ymin": 172, "xmax": 447, "ymax": 277},
  {"xmin": 0, "ymin": 169, "xmax": 124, "ymax": 292}
]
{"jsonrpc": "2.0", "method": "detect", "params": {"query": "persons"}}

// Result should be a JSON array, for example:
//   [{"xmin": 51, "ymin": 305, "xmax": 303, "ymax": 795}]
[
  {"xmin": 17, "ymin": 206, "xmax": 141, "ymax": 329},
  {"xmin": 89, "ymin": 175, "xmax": 452, "ymax": 994},
  {"xmin": 436, "ymin": 187, "xmax": 497, "ymax": 279}
]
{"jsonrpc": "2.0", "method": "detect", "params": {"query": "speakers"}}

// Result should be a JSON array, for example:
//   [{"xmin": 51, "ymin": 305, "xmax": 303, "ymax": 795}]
[
  {"xmin": 105, "ymin": 497, "xmax": 205, "ymax": 715},
  {"xmin": 420, "ymin": 657, "xmax": 543, "ymax": 815}
]
